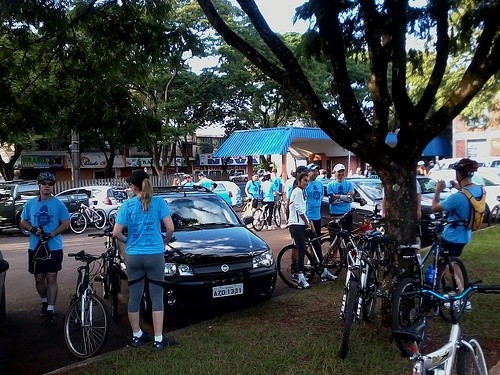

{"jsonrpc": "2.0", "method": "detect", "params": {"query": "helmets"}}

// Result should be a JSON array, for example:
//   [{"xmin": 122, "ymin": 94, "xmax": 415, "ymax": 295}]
[
  {"xmin": 455, "ymin": 158, "xmax": 477, "ymax": 172},
  {"xmin": 37, "ymin": 172, "xmax": 56, "ymax": 183}
]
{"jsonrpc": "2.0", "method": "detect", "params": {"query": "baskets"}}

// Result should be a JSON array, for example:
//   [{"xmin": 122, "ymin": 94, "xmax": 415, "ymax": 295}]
[{"xmin": 328, "ymin": 213, "xmax": 354, "ymax": 235}]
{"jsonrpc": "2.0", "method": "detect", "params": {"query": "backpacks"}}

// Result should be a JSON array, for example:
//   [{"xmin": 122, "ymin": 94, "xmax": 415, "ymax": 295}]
[{"xmin": 458, "ymin": 184, "xmax": 485, "ymax": 231}]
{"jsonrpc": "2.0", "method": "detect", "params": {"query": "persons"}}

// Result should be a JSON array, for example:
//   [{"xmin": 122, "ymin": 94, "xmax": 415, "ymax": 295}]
[
  {"xmin": 112, "ymin": 169, "xmax": 174, "ymax": 351},
  {"xmin": 245, "ymin": 168, "xmax": 297, "ymax": 230},
  {"xmin": 172, "ymin": 172, "xmax": 217, "ymax": 192},
  {"xmin": 380, "ymin": 177, "xmax": 422, "ymax": 267},
  {"xmin": 416, "ymin": 161, "xmax": 434, "ymax": 177},
  {"xmin": 286, "ymin": 172, "xmax": 338, "ymax": 289},
  {"xmin": 348, "ymin": 163, "xmax": 377, "ymax": 179},
  {"xmin": 20, "ymin": 172, "xmax": 71, "ymax": 327},
  {"xmin": 305, "ymin": 163, "xmax": 324, "ymax": 275},
  {"xmin": 327, "ymin": 163, "xmax": 356, "ymax": 266},
  {"xmin": 316, "ymin": 167, "xmax": 335, "ymax": 181},
  {"xmin": 432, "ymin": 158, "xmax": 486, "ymax": 313}
]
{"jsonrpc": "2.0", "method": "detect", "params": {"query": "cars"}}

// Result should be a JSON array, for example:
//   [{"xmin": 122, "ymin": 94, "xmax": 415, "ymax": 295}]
[
  {"xmin": 428, "ymin": 157, "xmax": 500, "ymax": 219},
  {"xmin": 229, "ymin": 171, "xmax": 249, "ymax": 182},
  {"xmin": 53, "ymin": 186, "xmax": 128, "ymax": 225},
  {"xmin": 319, "ymin": 177, "xmax": 454, "ymax": 237},
  {"xmin": 211, "ymin": 181, "xmax": 244, "ymax": 209}
]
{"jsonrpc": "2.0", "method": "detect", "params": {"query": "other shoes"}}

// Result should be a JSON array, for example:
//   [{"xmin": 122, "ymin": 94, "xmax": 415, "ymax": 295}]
[
  {"xmin": 444, "ymin": 298, "xmax": 471, "ymax": 312},
  {"xmin": 40, "ymin": 302, "xmax": 57, "ymax": 328},
  {"xmin": 297, "ymin": 272, "xmax": 311, "ymax": 288},
  {"xmin": 268, "ymin": 225, "xmax": 271, "ymax": 230},
  {"xmin": 132, "ymin": 332, "xmax": 152, "ymax": 347},
  {"xmin": 443, "ymin": 289, "xmax": 457, "ymax": 298},
  {"xmin": 321, "ymin": 267, "xmax": 338, "ymax": 281},
  {"xmin": 292, "ymin": 272, "xmax": 310, "ymax": 282},
  {"xmin": 152, "ymin": 337, "xmax": 176, "ymax": 351},
  {"xmin": 264, "ymin": 225, "xmax": 268, "ymax": 228}
]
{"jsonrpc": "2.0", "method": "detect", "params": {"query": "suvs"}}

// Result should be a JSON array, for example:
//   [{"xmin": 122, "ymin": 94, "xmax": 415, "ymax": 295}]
[
  {"xmin": 0, "ymin": 180, "xmax": 41, "ymax": 237},
  {"xmin": 114, "ymin": 184, "xmax": 278, "ymax": 321}
]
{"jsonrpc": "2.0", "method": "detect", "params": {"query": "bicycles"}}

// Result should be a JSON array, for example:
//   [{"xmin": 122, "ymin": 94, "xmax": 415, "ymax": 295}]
[
  {"xmin": 63, "ymin": 227, "xmax": 129, "ymax": 359},
  {"xmin": 69, "ymin": 199, "xmax": 120, "ymax": 234},
  {"xmin": 487, "ymin": 196, "xmax": 500, "ymax": 226},
  {"xmin": 388, "ymin": 280, "xmax": 500, "ymax": 375},
  {"xmin": 251, "ymin": 192, "xmax": 282, "ymax": 231},
  {"xmin": 276, "ymin": 204, "xmax": 470, "ymax": 360}
]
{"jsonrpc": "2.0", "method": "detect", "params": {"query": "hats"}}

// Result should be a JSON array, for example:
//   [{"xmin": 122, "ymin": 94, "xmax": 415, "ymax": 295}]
[
  {"xmin": 306, "ymin": 163, "xmax": 319, "ymax": 170},
  {"xmin": 125, "ymin": 169, "xmax": 149, "ymax": 186},
  {"xmin": 333, "ymin": 163, "xmax": 345, "ymax": 171},
  {"xmin": 198, "ymin": 171, "xmax": 206, "ymax": 176}
]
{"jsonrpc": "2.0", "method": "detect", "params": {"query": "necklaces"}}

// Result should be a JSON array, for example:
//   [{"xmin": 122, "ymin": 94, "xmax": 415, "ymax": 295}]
[{"xmin": 463, "ymin": 183, "xmax": 475, "ymax": 187}]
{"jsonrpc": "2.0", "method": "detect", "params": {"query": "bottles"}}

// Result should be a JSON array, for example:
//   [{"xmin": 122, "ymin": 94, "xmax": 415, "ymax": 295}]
[
  {"xmin": 433, "ymin": 365, "xmax": 445, "ymax": 375},
  {"xmin": 425, "ymin": 264, "xmax": 435, "ymax": 284}
]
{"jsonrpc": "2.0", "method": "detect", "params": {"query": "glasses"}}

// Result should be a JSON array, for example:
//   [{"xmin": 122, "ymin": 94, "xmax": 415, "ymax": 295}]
[{"xmin": 39, "ymin": 181, "xmax": 54, "ymax": 185}]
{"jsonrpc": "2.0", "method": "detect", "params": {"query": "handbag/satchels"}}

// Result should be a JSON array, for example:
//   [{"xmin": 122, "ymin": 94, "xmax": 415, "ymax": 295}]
[{"xmin": 252, "ymin": 197, "xmax": 258, "ymax": 207}]
